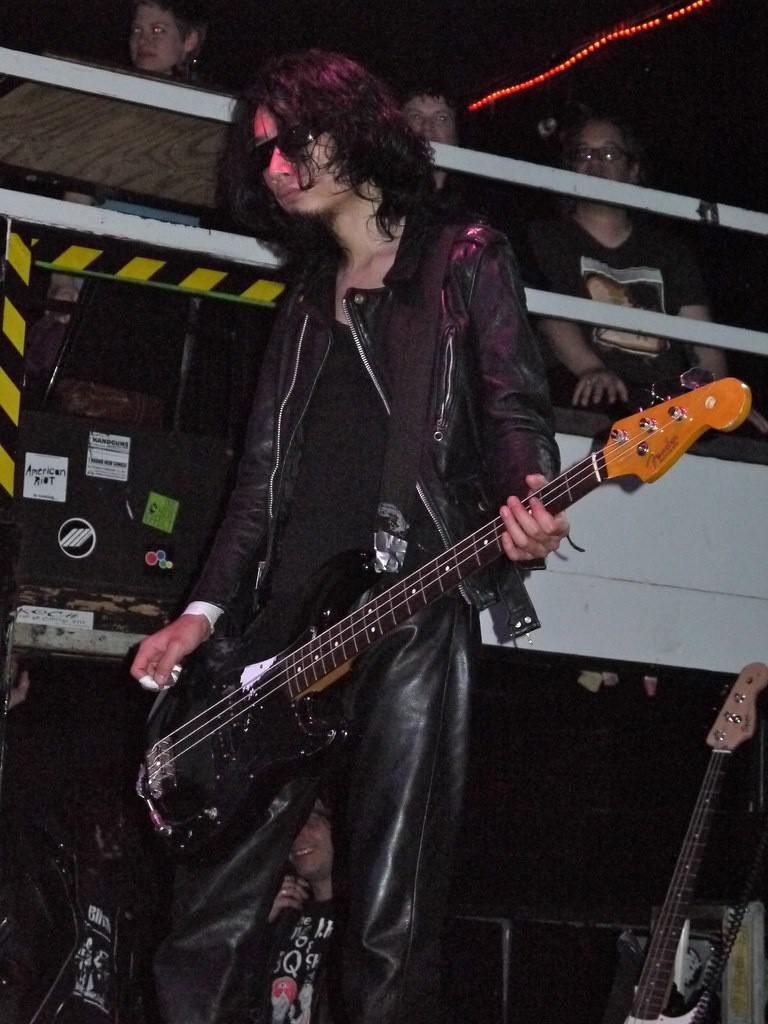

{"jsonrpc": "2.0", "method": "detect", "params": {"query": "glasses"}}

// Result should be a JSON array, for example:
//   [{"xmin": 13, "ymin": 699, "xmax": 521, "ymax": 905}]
[
  {"xmin": 250, "ymin": 117, "xmax": 312, "ymax": 172},
  {"xmin": 573, "ymin": 146, "xmax": 631, "ymax": 162},
  {"xmin": 305, "ymin": 811, "xmax": 332, "ymax": 825}
]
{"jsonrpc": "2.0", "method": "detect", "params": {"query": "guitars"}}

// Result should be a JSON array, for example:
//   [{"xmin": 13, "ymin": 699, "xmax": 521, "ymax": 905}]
[
  {"xmin": 597, "ymin": 662, "xmax": 768, "ymax": 1024},
  {"xmin": 120, "ymin": 374, "xmax": 752, "ymax": 864}
]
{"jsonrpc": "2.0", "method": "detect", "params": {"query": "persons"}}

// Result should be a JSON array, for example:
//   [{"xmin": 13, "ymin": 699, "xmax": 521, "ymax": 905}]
[
  {"xmin": 489, "ymin": 80, "xmax": 596, "ymax": 328},
  {"xmin": 527, "ymin": 109, "xmax": 768, "ymax": 441},
  {"xmin": 130, "ymin": 49, "xmax": 570, "ymax": 1023},
  {"xmin": 393, "ymin": 67, "xmax": 468, "ymax": 219},
  {"xmin": 0, "ymin": 372, "xmax": 238, "ymax": 1023},
  {"xmin": 257, "ymin": 783, "xmax": 351, "ymax": 1024},
  {"xmin": 44, "ymin": 0, "xmax": 208, "ymax": 394}
]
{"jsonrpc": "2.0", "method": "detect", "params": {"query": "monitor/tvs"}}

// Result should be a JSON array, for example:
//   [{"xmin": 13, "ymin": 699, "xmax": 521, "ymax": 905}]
[{"xmin": 15, "ymin": 408, "xmax": 227, "ymax": 596}]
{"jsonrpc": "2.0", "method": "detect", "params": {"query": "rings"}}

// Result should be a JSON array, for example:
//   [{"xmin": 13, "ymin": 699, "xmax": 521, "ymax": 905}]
[{"xmin": 280, "ymin": 888, "xmax": 288, "ymax": 897}]
